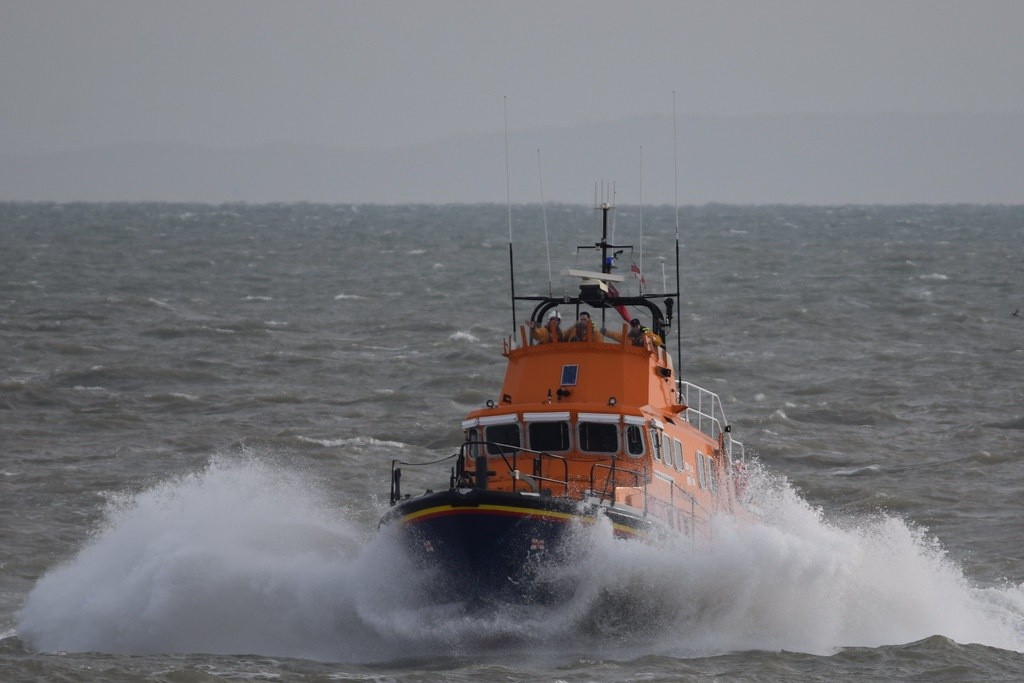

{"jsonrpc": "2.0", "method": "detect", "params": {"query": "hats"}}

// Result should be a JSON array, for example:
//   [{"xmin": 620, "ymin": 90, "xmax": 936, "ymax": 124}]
[{"xmin": 630, "ymin": 319, "xmax": 640, "ymax": 326}]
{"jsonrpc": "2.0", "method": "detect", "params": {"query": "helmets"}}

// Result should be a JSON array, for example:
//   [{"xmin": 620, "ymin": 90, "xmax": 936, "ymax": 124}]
[{"xmin": 547, "ymin": 310, "xmax": 562, "ymax": 324}]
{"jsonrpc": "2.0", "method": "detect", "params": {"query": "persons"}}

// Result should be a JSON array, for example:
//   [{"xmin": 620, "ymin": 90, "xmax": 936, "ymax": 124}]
[
  {"xmin": 525, "ymin": 311, "xmax": 563, "ymax": 344},
  {"xmin": 562, "ymin": 312, "xmax": 599, "ymax": 341},
  {"xmin": 600, "ymin": 319, "xmax": 662, "ymax": 346}
]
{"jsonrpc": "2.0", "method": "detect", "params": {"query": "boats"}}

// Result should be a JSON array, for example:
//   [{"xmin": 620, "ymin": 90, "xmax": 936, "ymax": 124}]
[{"xmin": 376, "ymin": 70, "xmax": 753, "ymax": 607}]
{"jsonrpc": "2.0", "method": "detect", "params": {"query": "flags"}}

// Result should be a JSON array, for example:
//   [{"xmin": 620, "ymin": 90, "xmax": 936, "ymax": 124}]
[
  {"xmin": 631, "ymin": 260, "xmax": 647, "ymax": 289},
  {"xmin": 607, "ymin": 283, "xmax": 632, "ymax": 322}
]
